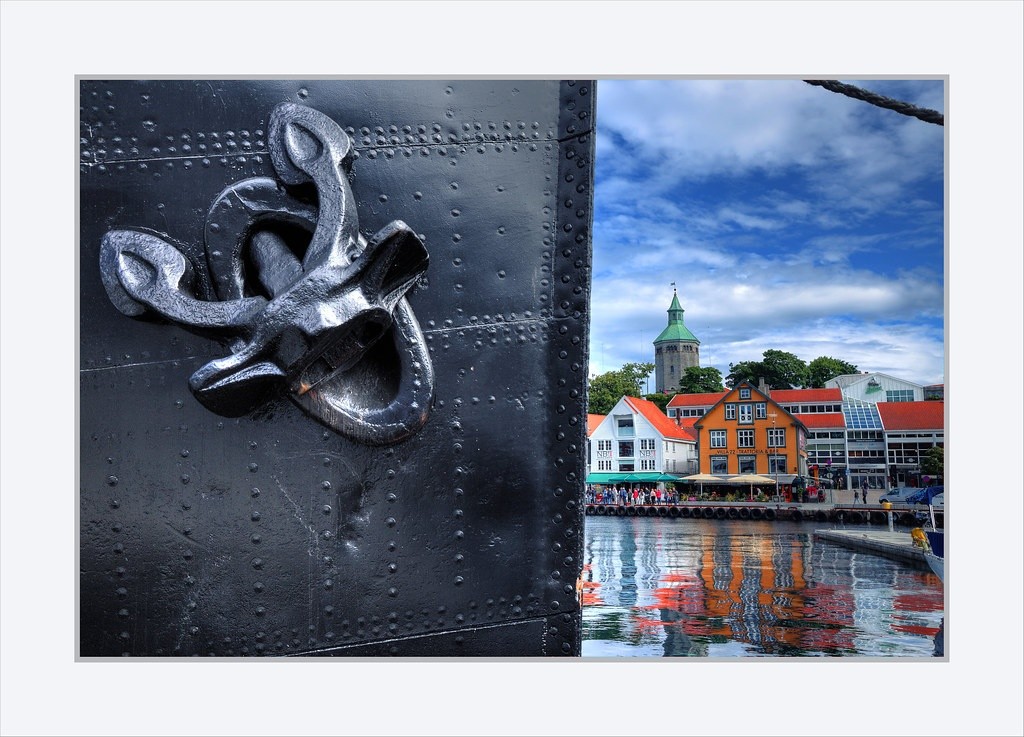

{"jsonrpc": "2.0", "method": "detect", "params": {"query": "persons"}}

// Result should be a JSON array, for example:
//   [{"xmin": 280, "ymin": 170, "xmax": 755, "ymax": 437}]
[
  {"xmin": 753, "ymin": 486, "xmax": 775, "ymax": 501},
  {"xmin": 678, "ymin": 486, "xmax": 720, "ymax": 501},
  {"xmin": 586, "ymin": 484, "xmax": 679, "ymax": 505},
  {"xmin": 911, "ymin": 527, "xmax": 929, "ymax": 554},
  {"xmin": 853, "ymin": 479, "xmax": 870, "ymax": 504},
  {"xmin": 817, "ymin": 472, "xmax": 846, "ymax": 489}
]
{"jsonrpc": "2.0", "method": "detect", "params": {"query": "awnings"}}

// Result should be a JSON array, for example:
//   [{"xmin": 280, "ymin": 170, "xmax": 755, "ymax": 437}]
[
  {"xmin": 586, "ymin": 472, "xmax": 689, "ymax": 484},
  {"xmin": 680, "ymin": 474, "xmax": 800, "ymax": 486}
]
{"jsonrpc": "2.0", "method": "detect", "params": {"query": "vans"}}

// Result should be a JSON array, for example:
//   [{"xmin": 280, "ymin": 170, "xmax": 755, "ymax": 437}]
[{"xmin": 879, "ymin": 487, "xmax": 924, "ymax": 504}]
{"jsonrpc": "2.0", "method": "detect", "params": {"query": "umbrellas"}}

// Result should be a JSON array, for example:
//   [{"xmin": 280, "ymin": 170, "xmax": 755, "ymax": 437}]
[
  {"xmin": 679, "ymin": 473, "xmax": 725, "ymax": 497},
  {"xmin": 727, "ymin": 472, "xmax": 776, "ymax": 501}
]
